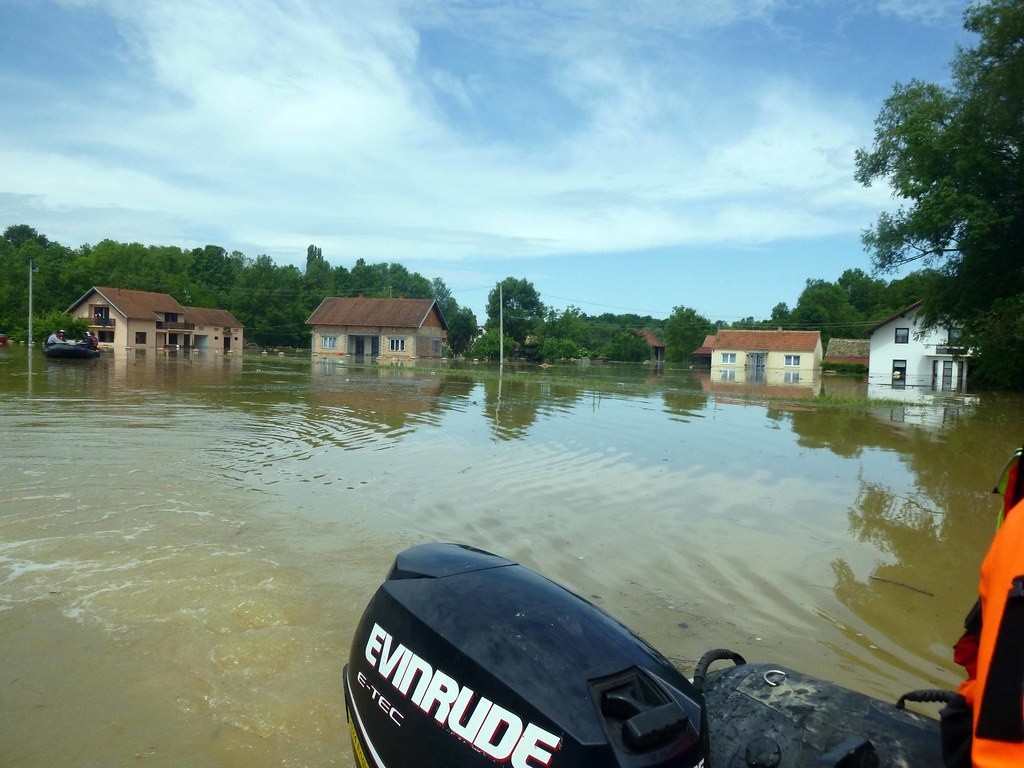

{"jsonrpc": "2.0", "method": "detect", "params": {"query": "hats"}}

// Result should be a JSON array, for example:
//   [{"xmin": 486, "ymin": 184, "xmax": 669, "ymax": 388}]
[{"xmin": 60, "ymin": 330, "xmax": 66, "ymax": 333}]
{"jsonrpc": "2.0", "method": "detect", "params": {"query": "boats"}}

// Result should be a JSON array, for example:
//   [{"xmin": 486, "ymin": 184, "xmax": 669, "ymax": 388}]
[
  {"xmin": 0, "ymin": 333, "xmax": 7, "ymax": 344},
  {"xmin": 42, "ymin": 342, "xmax": 100, "ymax": 357}
]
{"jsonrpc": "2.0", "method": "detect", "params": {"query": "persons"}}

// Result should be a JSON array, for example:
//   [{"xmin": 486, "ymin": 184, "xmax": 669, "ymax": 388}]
[
  {"xmin": 47, "ymin": 329, "xmax": 68, "ymax": 344},
  {"xmin": 97, "ymin": 313, "xmax": 103, "ymax": 318},
  {"xmin": 82, "ymin": 331, "xmax": 99, "ymax": 351}
]
